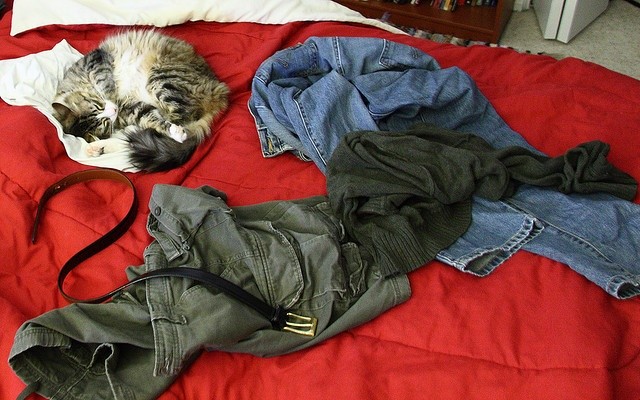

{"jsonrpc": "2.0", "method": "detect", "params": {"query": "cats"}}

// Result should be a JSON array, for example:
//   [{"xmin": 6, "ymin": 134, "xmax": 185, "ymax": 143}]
[{"xmin": 51, "ymin": 28, "xmax": 231, "ymax": 174}]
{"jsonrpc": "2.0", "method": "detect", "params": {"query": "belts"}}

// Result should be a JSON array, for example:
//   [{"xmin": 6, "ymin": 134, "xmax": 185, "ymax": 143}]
[{"xmin": 29, "ymin": 169, "xmax": 319, "ymax": 337}]
